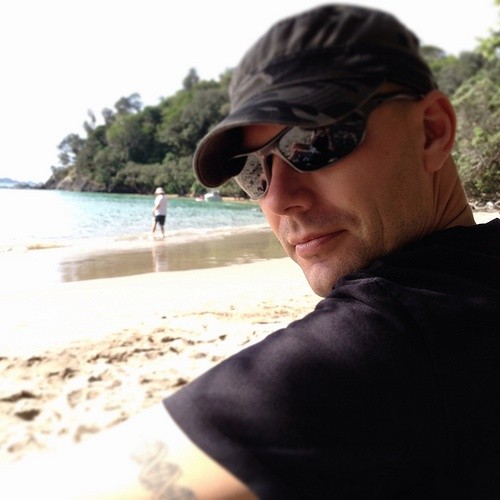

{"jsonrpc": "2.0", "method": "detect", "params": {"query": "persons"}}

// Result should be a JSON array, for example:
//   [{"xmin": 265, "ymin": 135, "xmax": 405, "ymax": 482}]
[
  {"xmin": 152, "ymin": 187, "xmax": 166, "ymax": 237},
  {"xmin": 161, "ymin": 3, "xmax": 500, "ymax": 499},
  {"xmin": 257, "ymin": 129, "xmax": 332, "ymax": 195}
]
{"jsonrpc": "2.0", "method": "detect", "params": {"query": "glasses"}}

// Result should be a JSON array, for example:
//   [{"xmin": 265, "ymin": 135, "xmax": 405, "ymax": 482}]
[{"xmin": 227, "ymin": 82, "xmax": 425, "ymax": 201}]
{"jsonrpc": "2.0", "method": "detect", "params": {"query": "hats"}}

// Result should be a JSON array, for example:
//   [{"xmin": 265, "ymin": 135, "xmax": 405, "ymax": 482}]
[
  {"xmin": 154, "ymin": 187, "xmax": 165, "ymax": 194},
  {"xmin": 190, "ymin": 3, "xmax": 436, "ymax": 190}
]
